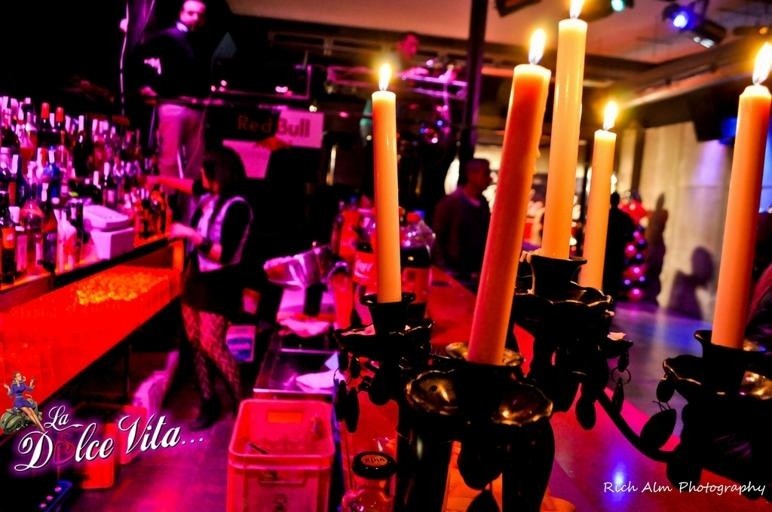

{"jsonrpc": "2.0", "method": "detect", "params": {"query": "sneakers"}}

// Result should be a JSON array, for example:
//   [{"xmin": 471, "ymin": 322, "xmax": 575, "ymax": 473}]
[{"xmin": 190, "ymin": 397, "xmax": 246, "ymax": 432}]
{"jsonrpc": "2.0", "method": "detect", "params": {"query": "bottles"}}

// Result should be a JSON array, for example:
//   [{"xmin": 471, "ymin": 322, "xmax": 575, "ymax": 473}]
[
  {"xmin": 338, "ymin": 450, "xmax": 396, "ymax": 512},
  {"xmin": 337, "ymin": 195, "xmax": 438, "ymax": 321},
  {"xmin": 0, "ymin": 94, "xmax": 166, "ymax": 284}
]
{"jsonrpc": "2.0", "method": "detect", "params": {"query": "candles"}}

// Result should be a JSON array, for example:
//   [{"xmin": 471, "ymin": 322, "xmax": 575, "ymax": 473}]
[{"xmin": 371, "ymin": 0, "xmax": 772, "ymax": 369}]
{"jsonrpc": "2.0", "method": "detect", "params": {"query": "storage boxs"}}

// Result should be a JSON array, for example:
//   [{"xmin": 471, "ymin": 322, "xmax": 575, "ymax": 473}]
[
  {"xmin": 225, "ymin": 399, "xmax": 336, "ymax": 512},
  {"xmin": 82, "ymin": 205, "xmax": 133, "ymax": 260}
]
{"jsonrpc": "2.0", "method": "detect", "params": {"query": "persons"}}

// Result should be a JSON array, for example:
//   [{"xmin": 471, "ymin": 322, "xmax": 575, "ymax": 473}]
[
  {"xmin": 430, "ymin": 156, "xmax": 493, "ymax": 298},
  {"xmin": 602, "ymin": 189, "xmax": 641, "ymax": 306},
  {"xmin": 128, "ymin": 1, "xmax": 235, "ymax": 219},
  {"xmin": 132, "ymin": 149, "xmax": 257, "ymax": 434}
]
